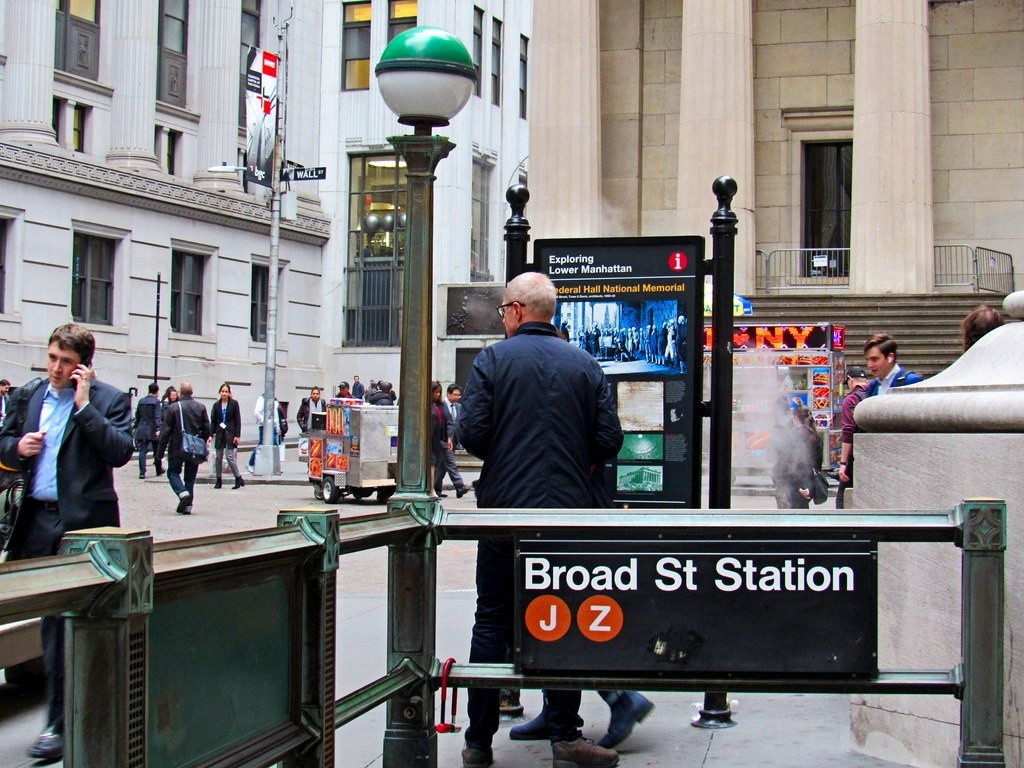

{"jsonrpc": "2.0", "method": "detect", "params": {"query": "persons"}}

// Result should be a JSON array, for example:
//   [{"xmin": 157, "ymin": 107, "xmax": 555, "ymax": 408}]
[
  {"xmin": 767, "ymin": 395, "xmax": 824, "ymax": 509},
  {"xmin": 961, "ymin": 306, "xmax": 1006, "ymax": 355},
  {"xmin": 864, "ymin": 333, "xmax": 926, "ymax": 399},
  {"xmin": 156, "ymin": 381, "xmax": 211, "ymax": 515},
  {"xmin": 334, "ymin": 381, "xmax": 356, "ymax": 398},
  {"xmin": 135, "ymin": 382, "xmax": 180, "ymax": 478},
  {"xmin": 509, "ymin": 689, "xmax": 655, "ymax": 749},
  {"xmin": 836, "ymin": 367, "xmax": 870, "ymax": 509},
  {"xmin": 456, "ymin": 272, "xmax": 626, "ymax": 768},
  {"xmin": 560, "ymin": 315, "xmax": 687, "ymax": 373},
  {"xmin": 0, "ymin": 379, "xmax": 11, "ymax": 432},
  {"xmin": 245, "ymin": 392, "xmax": 288, "ymax": 474},
  {"xmin": 365, "ymin": 379, "xmax": 397, "ymax": 406},
  {"xmin": 297, "ymin": 386, "xmax": 326, "ymax": 434},
  {"xmin": 352, "ymin": 375, "xmax": 364, "ymax": 399},
  {"xmin": 207, "ymin": 383, "xmax": 245, "ymax": 489},
  {"xmin": 0, "ymin": 323, "xmax": 134, "ymax": 758},
  {"xmin": 430, "ymin": 381, "xmax": 470, "ymax": 498}
]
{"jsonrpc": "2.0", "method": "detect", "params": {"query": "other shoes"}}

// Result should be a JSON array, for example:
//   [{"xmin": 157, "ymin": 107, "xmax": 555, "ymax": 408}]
[
  {"xmin": 181, "ymin": 511, "xmax": 191, "ymax": 515},
  {"xmin": 456, "ymin": 487, "xmax": 469, "ymax": 498},
  {"xmin": 176, "ymin": 495, "xmax": 192, "ymax": 513},
  {"xmin": 437, "ymin": 497, "xmax": 442, "ymax": 500},
  {"xmin": 460, "ymin": 740, "xmax": 494, "ymax": 768},
  {"xmin": 472, "ymin": 481, "xmax": 478, "ymax": 498},
  {"xmin": 509, "ymin": 711, "xmax": 583, "ymax": 741},
  {"xmin": 139, "ymin": 474, "xmax": 145, "ymax": 479},
  {"xmin": 438, "ymin": 491, "xmax": 447, "ymax": 497},
  {"xmin": 550, "ymin": 740, "xmax": 619, "ymax": 768},
  {"xmin": 245, "ymin": 462, "xmax": 253, "ymax": 472},
  {"xmin": 596, "ymin": 690, "xmax": 656, "ymax": 749},
  {"xmin": 27, "ymin": 729, "xmax": 65, "ymax": 757},
  {"xmin": 280, "ymin": 469, "xmax": 283, "ymax": 473},
  {"xmin": 156, "ymin": 468, "xmax": 166, "ymax": 476}
]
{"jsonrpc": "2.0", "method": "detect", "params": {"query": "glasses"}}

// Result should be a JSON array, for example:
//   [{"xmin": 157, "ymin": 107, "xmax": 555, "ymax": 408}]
[
  {"xmin": 495, "ymin": 300, "xmax": 526, "ymax": 317},
  {"xmin": 452, "ymin": 394, "xmax": 462, "ymax": 398}
]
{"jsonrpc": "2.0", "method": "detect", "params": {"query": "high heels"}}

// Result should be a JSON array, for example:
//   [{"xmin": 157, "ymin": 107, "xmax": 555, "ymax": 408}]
[
  {"xmin": 214, "ymin": 477, "xmax": 222, "ymax": 488},
  {"xmin": 232, "ymin": 475, "xmax": 246, "ymax": 489}
]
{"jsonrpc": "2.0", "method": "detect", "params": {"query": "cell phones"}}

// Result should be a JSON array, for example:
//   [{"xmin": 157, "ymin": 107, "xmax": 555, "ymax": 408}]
[{"xmin": 71, "ymin": 358, "xmax": 92, "ymax": 390}]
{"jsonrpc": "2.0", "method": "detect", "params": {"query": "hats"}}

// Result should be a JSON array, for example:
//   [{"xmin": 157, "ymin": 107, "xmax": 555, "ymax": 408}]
[
  {"xmin": 843, "ymin": 367, "xmax": 867, "ymax": 385},
  {"xmin": 338, "ymin": 382, "xmax": 349, "ymax": 388}
]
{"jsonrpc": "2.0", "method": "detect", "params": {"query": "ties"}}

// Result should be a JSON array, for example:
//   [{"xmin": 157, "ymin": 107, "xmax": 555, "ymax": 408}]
[{"xmin": 451, "ymin": 405, "xmax": 455, "ymax": 423}]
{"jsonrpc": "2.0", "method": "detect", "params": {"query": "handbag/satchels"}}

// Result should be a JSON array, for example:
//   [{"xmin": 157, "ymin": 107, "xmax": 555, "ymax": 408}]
[
  {"xmin": 278, "ymin": 443, "xmax": 286, "ymax": 461},
  {"xmin": 180, "ymin": 433, "xmax": 208, "ymax": 465},
  {"xmin": 813, "ymin": 471, "xmax": 830, "ymax": 505},
  {"xmin": 0, "ymin": 480, "xmax": 25, "ymax": 552},
  {"xmin": 280, "ymin": 419, "xmax": 288, "ymax": 433}
]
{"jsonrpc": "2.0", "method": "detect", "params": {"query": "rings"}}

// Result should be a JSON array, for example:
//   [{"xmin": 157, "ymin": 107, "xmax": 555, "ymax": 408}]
[{"xmin": 82, "ymin": 377, "xmax": 87, "ymax": 380}]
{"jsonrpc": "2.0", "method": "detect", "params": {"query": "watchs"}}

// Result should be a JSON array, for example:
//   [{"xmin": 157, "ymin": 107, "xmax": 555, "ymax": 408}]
[{"xmin": 840, "ymin": 462, "xmax": 849, "ymax": 466}]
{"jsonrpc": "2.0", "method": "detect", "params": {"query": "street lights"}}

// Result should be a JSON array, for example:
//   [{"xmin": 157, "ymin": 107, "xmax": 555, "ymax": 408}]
[
  {"xmin": 207, "ymin": 164, "xmax": 282, "ymax": 476},
  {"xmin": 373, "ymin": 28, "xmax": 481, "ymax": 767}
]
